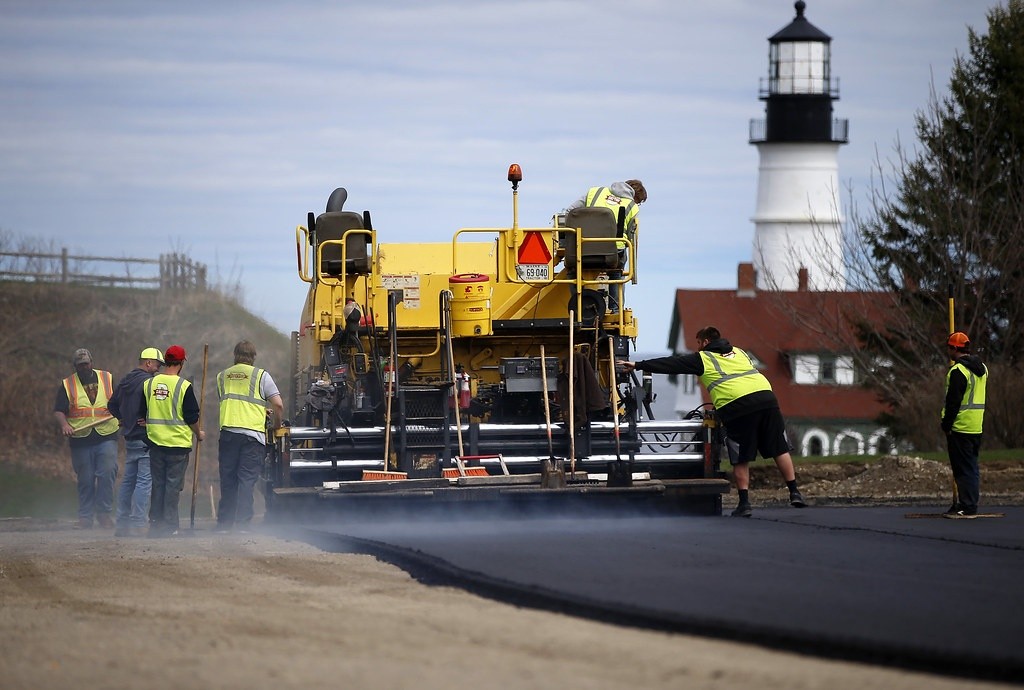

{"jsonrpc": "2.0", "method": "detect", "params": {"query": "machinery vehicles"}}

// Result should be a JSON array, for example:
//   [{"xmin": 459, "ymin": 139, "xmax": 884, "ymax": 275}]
[{"xmin": 261, "ymin": 162, "xmax": 735, "ymax": 528}]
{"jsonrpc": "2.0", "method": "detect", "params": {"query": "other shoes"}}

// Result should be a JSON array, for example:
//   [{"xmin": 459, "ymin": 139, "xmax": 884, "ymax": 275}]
[
  {"xmin": 74, "ymin": 517, "xmax": 93, "ymax": 529},
  {"xmin": 97, "ymin": 512, "xmax": 113, "ymax": 528},
  {"xmin": 115, "ymin": 529, "xmax": 129, "ymax": 537},
  {"xmin": 237, "ymin": 527, "xmax": 252, "ymax": 533},
  {"xmin": 151, "ymin": 520, "xmax": 178, "ymax": 534},
  {"xmin": 215, "ymin": 528, "xmax": 231, "ymax": 534},
  {"xmin": 131, "ymin": 528, "xmax": 147, "ymax": 536}
]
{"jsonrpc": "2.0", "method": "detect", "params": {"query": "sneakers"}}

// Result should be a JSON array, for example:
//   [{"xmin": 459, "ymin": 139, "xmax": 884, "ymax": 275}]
[
  {"xmin": 732, "ymin": 502, "xmax": 752, "ymax": 517},
  {"xmin": 790, "ymin": 492, "xmax": 807, "ymax": 507},
  {"xmin": 943, "ymin": 504, "xmax": 978, "ymax": 518}
]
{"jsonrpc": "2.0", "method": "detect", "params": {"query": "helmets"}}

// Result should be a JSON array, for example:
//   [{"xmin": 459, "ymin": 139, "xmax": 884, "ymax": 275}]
[{"xmin": 140, "ymin": 348, "xmax": 166, "ymax": 366}]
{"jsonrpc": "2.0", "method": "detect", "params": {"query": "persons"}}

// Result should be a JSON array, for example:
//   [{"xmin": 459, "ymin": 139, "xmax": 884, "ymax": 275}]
[
  {"xmin": 942, "ymin": 332, "xmax": 988, "ymax": 519},
  {"xmin": 53, "ymin": 350, "xmax": 120, "ymax": 529},
  {"xmin": 216, "ymin": 340, "xmax": 284, "ymax": 530},
  {"xmin": 564, "ymin": 179, "xmax": 647, "ymax": 315},
  {"xmin": 107, "ymin": 348, "xmax": 165, "ymax": 537},
  {"xmin": 139, "ymin": 345, "xmax": 205, "ymax": 540},
  {"xmin": 616, "ymin": 327, "xmax": 805, "ymax": 517}
]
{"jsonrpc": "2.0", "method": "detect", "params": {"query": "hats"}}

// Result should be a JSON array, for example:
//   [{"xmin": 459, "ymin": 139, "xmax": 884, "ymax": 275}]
[
  {"xmin": 946, "ymin": 332, "xmax": 971, "ymax": 348},
  {"xmin": 165, "ymin": 346, "xmax": 187, "ymax": 361},
  {"xmin": 72, "ymin": 348, "xmax": 92, "ymax": 366}
]
